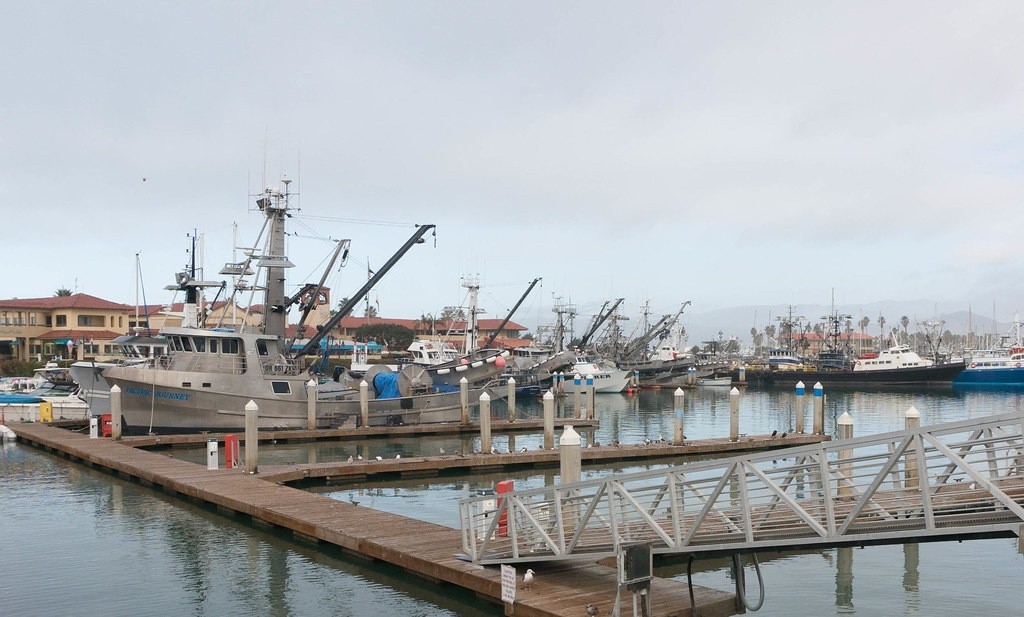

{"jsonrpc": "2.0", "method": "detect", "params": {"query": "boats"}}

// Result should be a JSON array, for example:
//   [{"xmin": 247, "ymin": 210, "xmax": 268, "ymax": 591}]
[
  {"xmin": 730, "ymin": 301, "xmax": 967, "ymax": 384},
  {"xmin": 768, "ymin": 305, "xmax": 805, "ymax": 370},
  {"xmin": 0, "ymin": 376, "xmax": 45, "ymax": 394},
  {"xmin": 348, "ymin": 255, "xmax": 542, "ymax": 396},
  {"xmin": 694, "ymin": 338, "xmax": 736, "ymax": 378},
  {"xmin": 102, "ymin": 123, "xmax": 533, "ymax": 432},
  {"xmin": 956, "ymin": 311, "xmax": 1024, "ymax": 383},
  {"xmin": 697, "ymin": 376, "xmax": 732, "ymax": 386},
  {"xmin": 814, "ymin": 309, "xmax": 853, "ymax": 371},
  {"xmin": 618, "ymin": 301, "xmax": 694, "ymax": 389},
  {"xmin": 555, "ymin": 297, "xmax": 629, "ymax": 394},
  {"xmin": 0, "ymin": 364, "xmax": 92, "ymax": 422},
  {"xmin": 506, "ymin": 293, "xmax": 577, "ymax": 384},
  {"xmin": 69, "ymin": 228, "xmax": 227, "ymax": 419}
]
